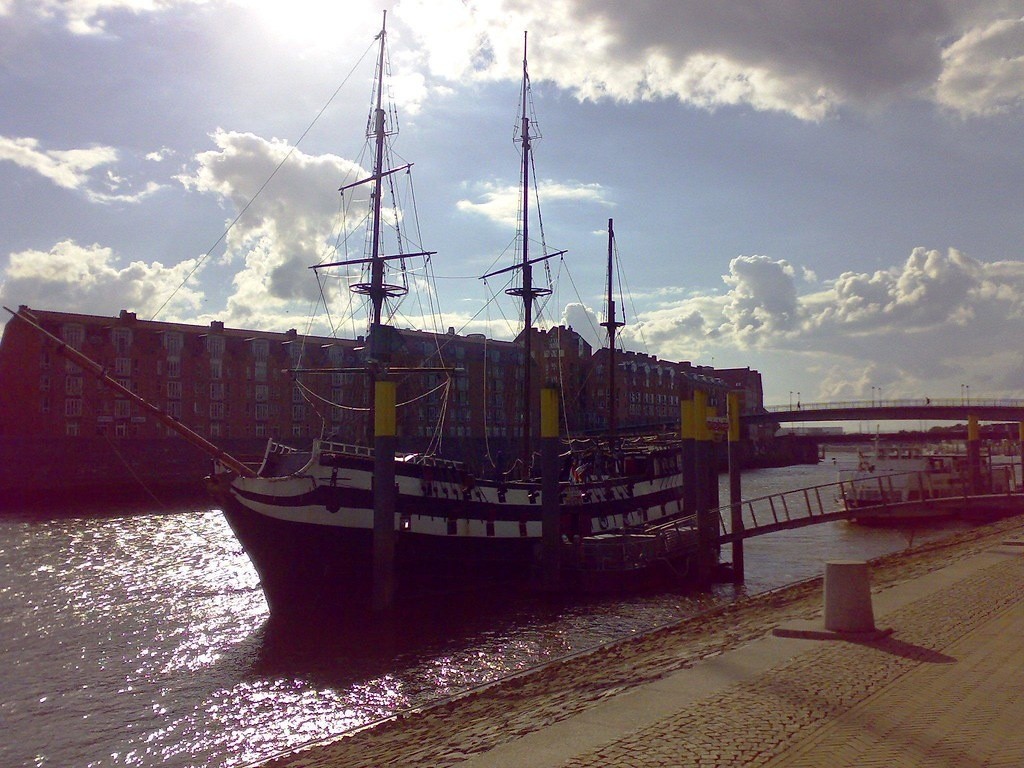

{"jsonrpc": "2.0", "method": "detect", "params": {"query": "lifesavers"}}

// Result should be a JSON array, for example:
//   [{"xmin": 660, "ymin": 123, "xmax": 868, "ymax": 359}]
[{"xmin": 859, "ymin": 461, "xmax": 870, "ymax": 471}]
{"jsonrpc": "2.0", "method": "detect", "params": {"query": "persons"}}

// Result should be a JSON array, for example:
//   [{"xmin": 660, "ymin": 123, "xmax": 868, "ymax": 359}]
[
  {"xmin": 926, "ymin": 397, "xmax": 930, "ymax": 407},
  {"xmin": 797, "ymin": 400, "xmax": 800, "ymax": 411}
]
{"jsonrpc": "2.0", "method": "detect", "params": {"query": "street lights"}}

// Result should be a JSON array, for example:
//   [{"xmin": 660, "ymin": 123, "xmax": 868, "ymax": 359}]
[
  {"xmin": 872, "ymin": 387, "xmax": 875, "ymax": 407},
  {"xmin": 797, "ymin": 392, "xmax": 800, "ymax": 411},
  {"xmin": 966, "ymin": 386, "xmax": 969, "ymax": 405},
  {"xmin": 878, "ymin": 388, "xmax": 882, "ymax": 409},
  {"xmin": 961, "ymin": 384, "xmax": 964, "ymax": 405},
  {"xmin": 790, "ymin": 391, "xmax": 793, "ymax": 412}
]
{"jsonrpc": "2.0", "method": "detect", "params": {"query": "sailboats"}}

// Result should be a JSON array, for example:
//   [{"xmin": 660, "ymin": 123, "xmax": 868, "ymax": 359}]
[{"xmin": 3, "ymin": 8, "xmax": 684, "ymax": 615}]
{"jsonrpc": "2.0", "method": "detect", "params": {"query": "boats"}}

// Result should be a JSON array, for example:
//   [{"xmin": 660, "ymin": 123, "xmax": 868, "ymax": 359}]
[{"xmin": 833, "ymin": 424, "xmax": 1011, "ymax": 525}]
